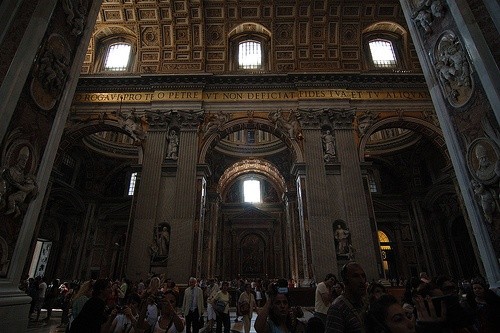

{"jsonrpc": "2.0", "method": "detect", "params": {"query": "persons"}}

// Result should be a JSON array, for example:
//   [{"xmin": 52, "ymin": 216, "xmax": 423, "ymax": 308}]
[
  {"xmin": 323, "ymin": 130, "xmax": 337, "ymax": 162},
  {"xmin": 411, "ymin": 1, "xmax": 471, "ymax": 99},
  {"xmin": 166, "ymin": 128, "xmax": 179, "ymax": 158},
  {"xmin": 471, "ymin": 145, "xmax": 500, "ymax": 225},
  {"xmin": 156, "ymin": 227, "xmax": 169, "ymax": 255},
  {"xmin": 22, "ymin": 265, "xmax": 500, "ymax": 333},
  {"xmin": 0, "ymin": 5, "xmax": 89, "ymax": 219},
  {"xmin": 336, "ymin": 224, "xmax": 350, "ymax": 254}
]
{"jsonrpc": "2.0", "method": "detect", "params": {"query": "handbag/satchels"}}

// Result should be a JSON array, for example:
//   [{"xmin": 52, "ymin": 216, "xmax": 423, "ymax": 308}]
[
  {"xmin": 236, "ymin": 303, "xmax": 252, "ymax": 315},
  {"xmin": 213, "ymin": 301, "xmax": 226, "ymax": 313}
]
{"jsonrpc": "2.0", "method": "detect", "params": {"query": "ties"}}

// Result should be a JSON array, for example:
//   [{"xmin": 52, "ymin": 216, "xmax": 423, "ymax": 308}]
[{"xmin": 190, "ymin": 288, "xmax": 194, "ymax": 309}]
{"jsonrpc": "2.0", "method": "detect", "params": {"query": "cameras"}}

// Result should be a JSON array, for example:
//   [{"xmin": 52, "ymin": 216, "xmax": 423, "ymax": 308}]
[
  {"xmin": 273, "ymin": 278, "xmax": 289, "ymax": 295},
  {"xmin": 413, "ymin": 293, "xmax": 470, "ymax": 333},
  {"xmin": 116, "ymin": 298, "xmax": 128, "ymax": 314}
]
